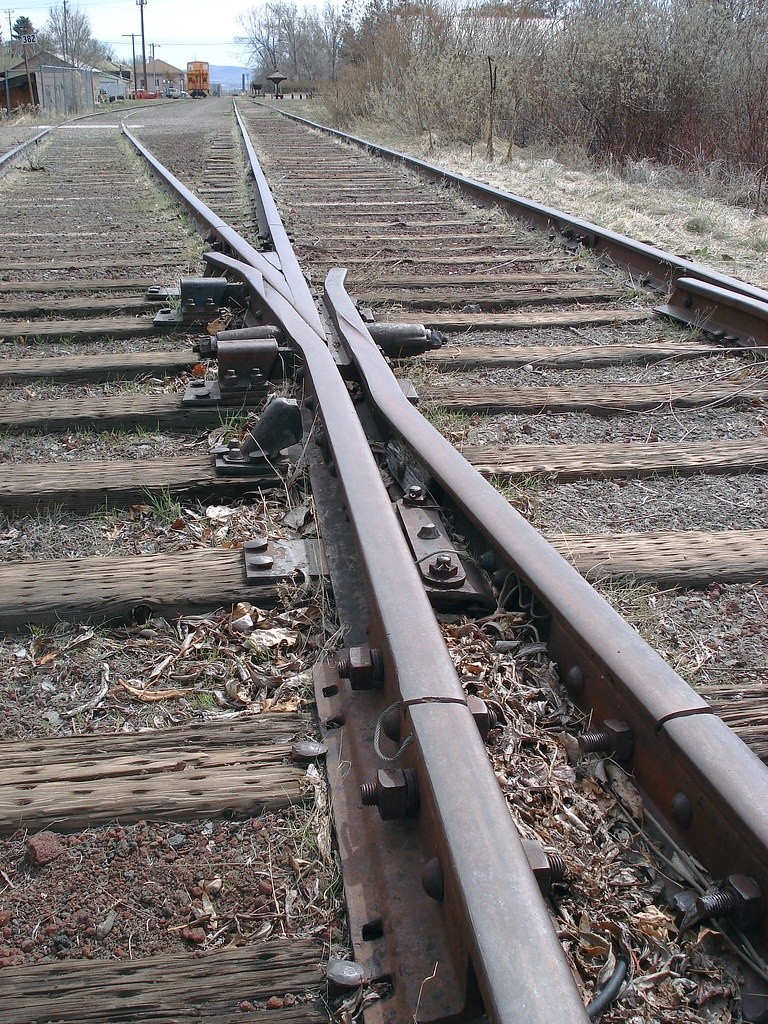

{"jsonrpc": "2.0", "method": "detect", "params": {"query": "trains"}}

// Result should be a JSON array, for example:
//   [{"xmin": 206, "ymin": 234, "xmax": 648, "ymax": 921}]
[{"xmin": 187, "ymin": 60, "xmax": 211, "ymax": 98}]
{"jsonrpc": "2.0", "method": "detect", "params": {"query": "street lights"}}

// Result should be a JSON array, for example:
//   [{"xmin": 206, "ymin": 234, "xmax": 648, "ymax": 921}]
[{"xmin": 136, "ymin": 0, "xmax": 147, "ymax": 91}]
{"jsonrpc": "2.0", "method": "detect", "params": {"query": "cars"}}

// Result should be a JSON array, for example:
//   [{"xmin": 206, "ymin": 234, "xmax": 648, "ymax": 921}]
[
  {"xmin": 160, "ymin": 88, "xmax": 181, "ymax": 98},
  {"xmin": 131, "ymin": 89, "xmax": 155, "ymax": 99}
]
